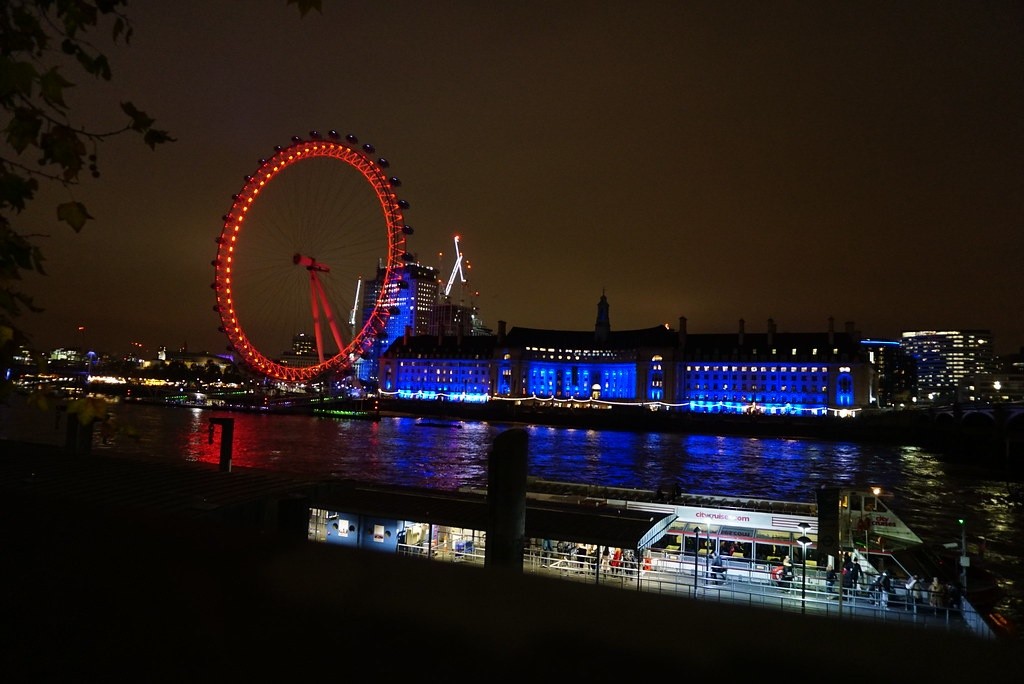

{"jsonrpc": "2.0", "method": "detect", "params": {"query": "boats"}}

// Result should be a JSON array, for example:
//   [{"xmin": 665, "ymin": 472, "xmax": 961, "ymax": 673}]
[
  {"xmin": 416, "ymin": 415, "xmax": 450, "ymax": 427},
  {"xmin": 310, "ymin": 399, "xmax": 382, "ymax": 422}
]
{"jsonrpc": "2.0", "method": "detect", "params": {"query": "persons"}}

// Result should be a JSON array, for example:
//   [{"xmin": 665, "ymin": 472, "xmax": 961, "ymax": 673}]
[
  {"xmin": 880, "ymin": 568, "xmax": 890, "ymax": 610},
  {"xmin": 711, "ymin": 553, "xmax": 722, "ymax": 584},
  {"xmin": 929, "ymin": 578, "xmax": 961, "ymax": 615},
  {"xmin": 781, "ymin": 556, "xmax": 793, "ymax": 592},
  {"xmin": 826, "ymin": 555, "xmax": 861, "ymax": 599},
  {"xmin": 541, "ymin": 539, "xmax": 636, "ymax": 581}
]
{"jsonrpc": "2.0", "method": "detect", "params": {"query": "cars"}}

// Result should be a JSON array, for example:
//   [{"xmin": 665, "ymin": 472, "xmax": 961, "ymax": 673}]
[
  {"xmin": 865, "ymin": 577, "xmax": 923, "ymax": 606},
  {"xmin": 931, "ymin": 402, "xmax": 948, "ymax": 411},
  {"xmin": 752, "ymin": 413, "xmax": 768, "ymax": 421},
  {"xmin": 912, "ymin": 401, "xmax": 930, "ymax": 410},
  {"xmin": 971, "ymin": 397, "xmax": 992, "ymax": 409},
  {"xmin": 724, "ymin": 417, "xmax": 734, "ymax": 423}
]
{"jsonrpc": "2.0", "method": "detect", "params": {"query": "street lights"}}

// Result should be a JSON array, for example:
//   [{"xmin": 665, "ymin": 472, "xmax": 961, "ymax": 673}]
[
  {"xmin": 871, "ymin": 485, "xmax": 881, "ymax": 511},
  {"xmin": 694, "ymin": 527, "xmax": 702, "ymax": 599},
  {"xmin": 796, "ymin": 523, "xmax": 813, "ymax": 613}
]
